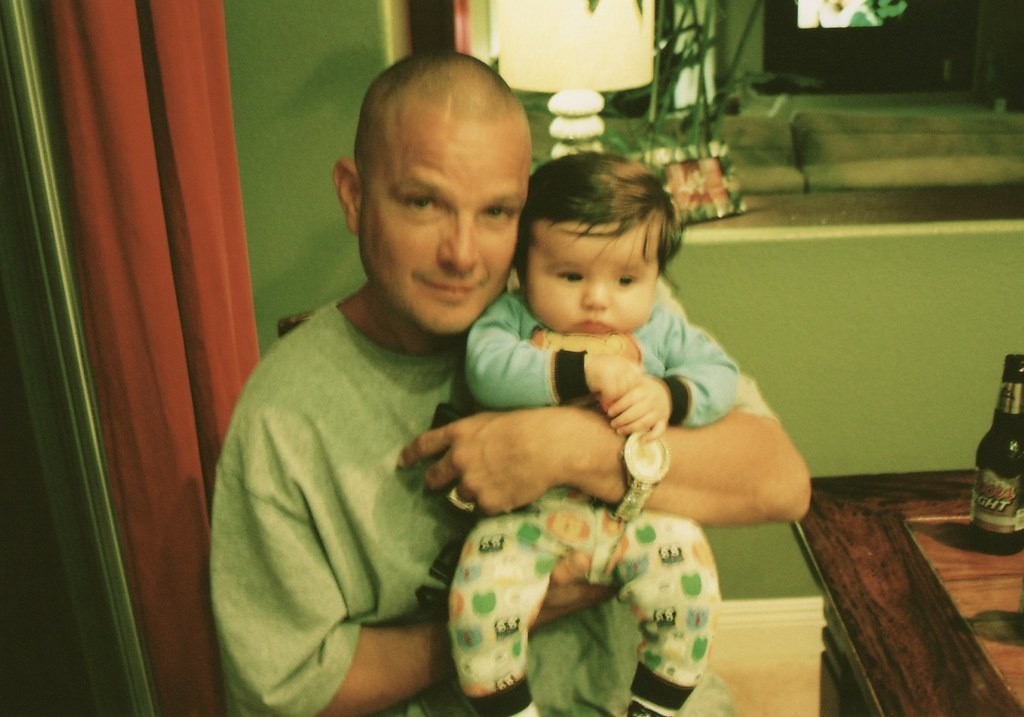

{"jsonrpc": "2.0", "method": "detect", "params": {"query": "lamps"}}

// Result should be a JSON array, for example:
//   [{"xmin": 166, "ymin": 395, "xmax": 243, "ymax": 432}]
[{"xmin": 491, "ymin": 1, "xmax": 653, "ymax": 170}]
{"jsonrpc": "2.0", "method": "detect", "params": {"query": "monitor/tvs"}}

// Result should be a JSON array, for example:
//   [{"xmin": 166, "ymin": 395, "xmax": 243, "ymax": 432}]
[{"xmin": 725, "ymin": 0, "xmax": 981, "ymax": 106}]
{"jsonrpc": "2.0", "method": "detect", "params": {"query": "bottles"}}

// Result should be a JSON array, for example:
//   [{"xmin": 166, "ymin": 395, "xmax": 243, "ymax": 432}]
[{"xmin": 969, "ymin": 354, "xmax": 1024, "ymax": 553}]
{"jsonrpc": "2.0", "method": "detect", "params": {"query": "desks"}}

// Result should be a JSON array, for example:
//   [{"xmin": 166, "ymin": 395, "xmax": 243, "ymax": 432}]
[{"xmin": 799, "ymin": 466, "xmax": 1023, "ymax": 717}]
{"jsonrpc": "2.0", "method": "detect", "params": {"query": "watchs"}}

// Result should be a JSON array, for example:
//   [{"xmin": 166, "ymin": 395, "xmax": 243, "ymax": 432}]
[{"xmin": 614, "ymin": 430, "xmax": 668, "ymax": 520}]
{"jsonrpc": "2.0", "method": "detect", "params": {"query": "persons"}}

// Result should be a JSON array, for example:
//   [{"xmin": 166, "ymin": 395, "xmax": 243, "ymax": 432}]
[
  {"xmin": 212, "ymin": 51, "xmax": 812, "ymax": 717},
  {"xmin": 450, "ymin": 152, "xmax": 737, "ymax": 716}
]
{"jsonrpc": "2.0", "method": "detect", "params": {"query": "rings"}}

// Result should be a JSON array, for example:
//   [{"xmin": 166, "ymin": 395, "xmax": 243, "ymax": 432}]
[{"xmin": 447, "ymin": 488, "xmax": 474, "ymax": 513}]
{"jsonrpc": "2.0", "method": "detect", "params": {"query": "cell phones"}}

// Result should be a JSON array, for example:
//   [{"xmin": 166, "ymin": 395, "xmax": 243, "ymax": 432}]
[{"xmin": 428, "ymin": 403, "xmax": 532, "ymax": 513}]
